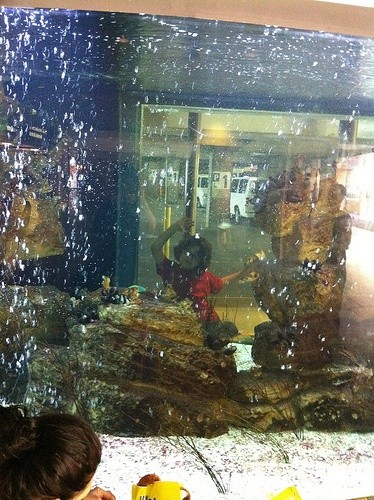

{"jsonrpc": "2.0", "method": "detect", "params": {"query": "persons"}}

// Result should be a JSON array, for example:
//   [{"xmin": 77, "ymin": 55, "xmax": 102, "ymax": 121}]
[
  {"xmin": 326, "ymin": 184, "xmax": 353, "ymax": 330},
  {"xmin": 0, "ymin": 403, "xmax": 121, "ymax": 500},
  {"xmin": 138, "ymin": 181, "xmax": 159, "ymax": 236},
  {"xmin": 152, "ymin": 216, "xmax": 257, "ymax": 328}
]
